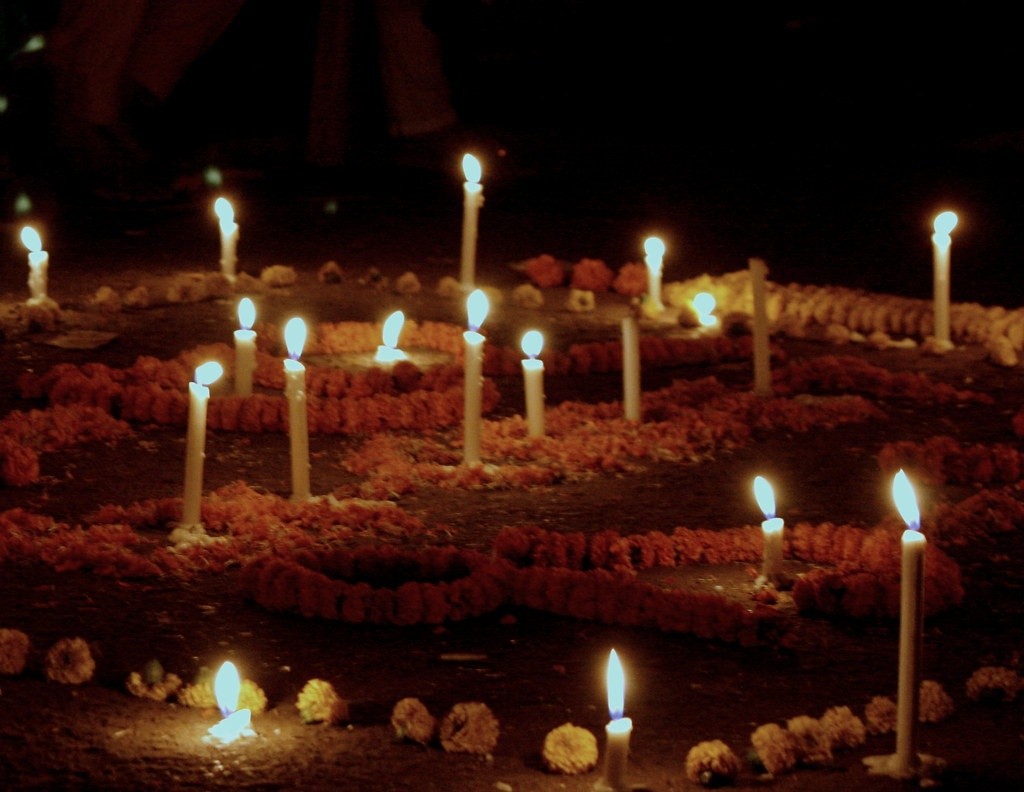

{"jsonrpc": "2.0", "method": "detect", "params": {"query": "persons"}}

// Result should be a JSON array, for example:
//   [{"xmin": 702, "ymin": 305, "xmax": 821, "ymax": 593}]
[{"xmin": 44, "ymin": 0, "xmax": 243, "ymax": 235}]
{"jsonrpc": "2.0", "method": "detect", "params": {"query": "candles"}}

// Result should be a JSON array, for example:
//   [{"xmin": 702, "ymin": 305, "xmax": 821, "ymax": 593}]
[
  {"xmin": 750, "ymin": 259, "xmax": 772, "ymax": 392},
  {"xmin": 233, "ymin": 297, "xmax": 259, "ymax": 395},
  {"xmin": 214, "ymin": 197, "xmax": 239, "ymax": 280},
  {"xmin": 606, "ymin": 647, "xmax": 634, "ymax": 789},
  {"xmin": 209, "ymin": 659, "xmax": 250, "ymax": 743},
  {"xmin": 20, "ymin": 225, "xmax": 49, "ymax": 297},
  {"xmin": 752, "ymin": 475, "xmax": 784, "ymax": 579},
  {"xmin": 282, "ymin": 317, "xmax": 310, "ymax": 500},
  {"xmin": 643, "ymin": 236, "xmax": 667, "ymax": 310},
  {"xmin": 375, "ymin": 310, "xmax": 406, "ymax": 364},
  {"xmin": 892, "ymin": 468, "xmax": 927, "ymax": 774},
  {"xmin": 931, "ymin": 212, "xmax": 959, "ymax": 344},
  {"xmin": 461, "ymin": 152, "xmax": 483, "ymax": 288},
  {"xmin": 520, "ymin": 329, "xmax": 546, "ymax": 435},
  {"xmin": 624, "ymin": 317, "xmax": 641, "ymax": 422},
  {"xmin": 462, "ymin": 288, "xmax": 490, "ymax": 466},
  {"xmin": 181, "ymin": 360, "xmax": 223, "ymax": 524}
]
